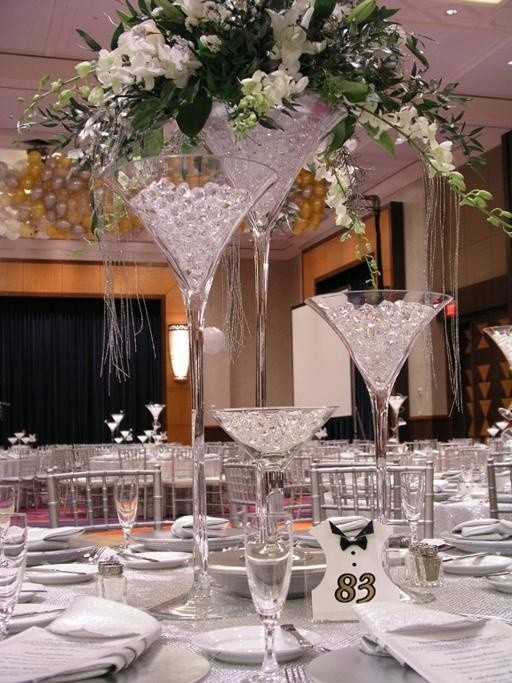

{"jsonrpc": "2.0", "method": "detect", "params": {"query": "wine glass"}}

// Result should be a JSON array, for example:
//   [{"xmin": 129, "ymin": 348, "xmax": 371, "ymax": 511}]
[
  {"xmin": 207, "ymin": 405, "xmax": 338, "ymax": 540},
  {"xmin": 106, "ymin": 404, "xmax": 173, "ymax": 448},
  {"xmin": 115, "ymin": 478, "xmax": 138, "ymax": 553},
  {"xmin": 9, "ymin": 428, "xmax": 37, "ymax": 455},
  {"xmin": 414, "ymin": 436, "xmax": 512, "ymax": 505},
  {"xmin": 399, "ymin": 472, "xmax": 424, "ymax": 543},
  {"xmin": 245, "ymin": 509, "xmax": 294, "ymax": 664},
  {"xmin": 303, "ymin": 289, "xmax": 454, "ymax": 520},
  {"xmin": 101, "ymin": 153, "xmax": 278, "ymax": 621}
]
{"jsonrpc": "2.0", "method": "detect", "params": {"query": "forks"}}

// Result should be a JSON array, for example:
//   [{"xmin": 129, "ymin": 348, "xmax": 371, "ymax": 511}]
[
  {"xmin": 283, "ymin": 665, "xmax": 310, "ymax": 682},
  {"xmin": 281, "ymin": 623, "xmax": 317, "ymax": 658}
]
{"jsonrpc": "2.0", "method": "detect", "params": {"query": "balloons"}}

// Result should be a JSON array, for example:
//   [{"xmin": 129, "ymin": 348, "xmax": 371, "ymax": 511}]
[{"xmin": 0, "ymin": 148, "xmax": 333, "ymax": 246}]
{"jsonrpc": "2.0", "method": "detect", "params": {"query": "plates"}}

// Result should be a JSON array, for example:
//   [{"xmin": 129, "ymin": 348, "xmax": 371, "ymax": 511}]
[
  {"xmin": 107, "ymin": 641, "xmax": 212, "ymax": 682},
  {"xmin": 126, "ymin": 551, "xmax": 193, "ymax": 569},
  {"xmin": 9, "ymin": 526, "xmax": 100, "ymax": 632},
  {"xmin": 193, "ymin": 626, "xmax": 322, "ymax": 666},
  {"xmin": 442, "ymin": 512, "xmax": 512, "ymax": 592}
]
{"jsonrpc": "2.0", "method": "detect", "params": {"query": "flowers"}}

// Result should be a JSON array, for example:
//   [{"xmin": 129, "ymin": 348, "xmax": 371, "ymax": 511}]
[{"xmin": 8, "ymin": 0, "xmax": 512, "ymax": 287}]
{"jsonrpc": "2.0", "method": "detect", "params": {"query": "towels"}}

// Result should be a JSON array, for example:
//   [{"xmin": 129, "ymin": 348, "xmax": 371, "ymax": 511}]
[
  {"xmin": 41, "ymin": 597, "xmax": 165, "ymax": 683},
  {"xmin": 24, "ymin": 527, "xmax": 85, "ymax": 551},
  {"xmin": 353, "ymin": 601, "xmax": 485, "ymax": 666}
]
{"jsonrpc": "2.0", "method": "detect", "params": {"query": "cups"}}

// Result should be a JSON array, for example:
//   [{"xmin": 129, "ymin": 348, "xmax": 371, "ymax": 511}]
[{"xmin": 0, "ymin": 513, "xmax": 29, "ymax": 637}]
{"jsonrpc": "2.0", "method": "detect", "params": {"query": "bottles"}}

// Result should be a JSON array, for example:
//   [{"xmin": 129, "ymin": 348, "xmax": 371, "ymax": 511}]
[
  {"xmin": 95, "ymin": 561, "xmax": 127, "ymax": 603},
  {"xmin": 407, "ymin": 543, "xmax": 439, "ymax": 584}
]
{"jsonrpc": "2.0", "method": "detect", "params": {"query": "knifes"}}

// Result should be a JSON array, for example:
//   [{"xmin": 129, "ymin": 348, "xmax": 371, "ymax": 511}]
[{"xmin": 117, "ymin": 551, "xmax": 158, "ymax": 562}]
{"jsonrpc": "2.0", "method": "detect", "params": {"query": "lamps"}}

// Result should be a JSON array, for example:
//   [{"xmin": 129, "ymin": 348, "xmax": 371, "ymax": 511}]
[{"xmin": 167, "ymin": 323, "xmax": 189, "ymax": 382}]
